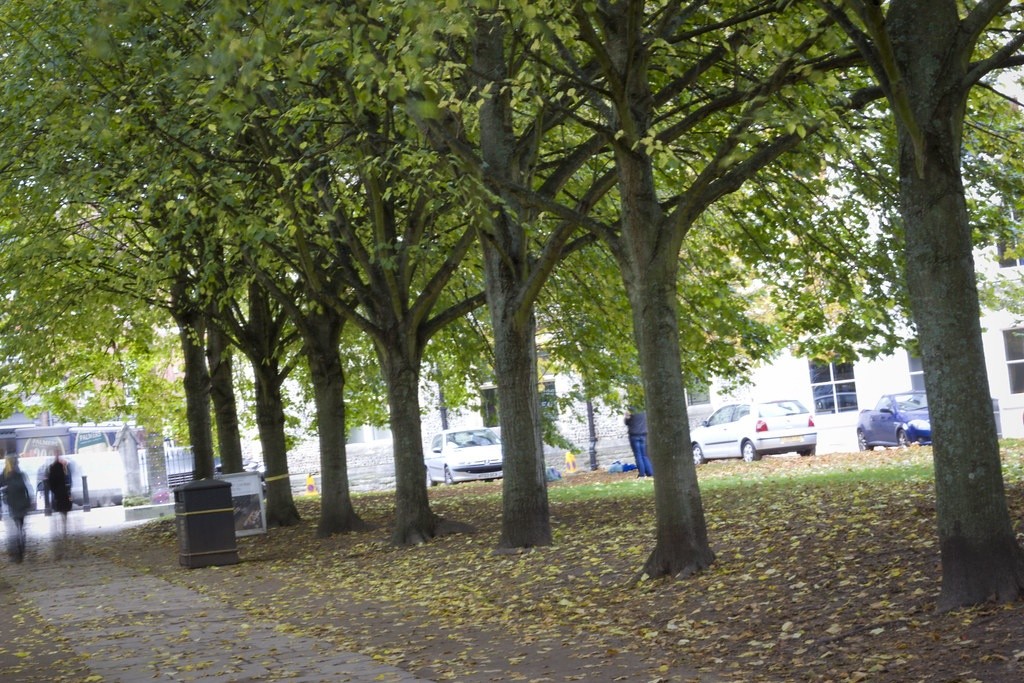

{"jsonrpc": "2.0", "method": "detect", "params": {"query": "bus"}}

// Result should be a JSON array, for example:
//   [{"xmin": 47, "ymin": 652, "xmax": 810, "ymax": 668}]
[{"xmin": 1, "ymin": 425, "xmax": 146, "ymax": 505}]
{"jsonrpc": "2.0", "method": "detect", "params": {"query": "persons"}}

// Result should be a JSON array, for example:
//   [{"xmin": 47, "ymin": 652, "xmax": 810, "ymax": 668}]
[
  {"xmin": 0, "ymin": 454, "xmax": 31, "ymax": 563},
  {"xmin": 624, "ymin": 397, "xmax": 653, "ymax": 478},
  {"xmin": 47, "ymin": 452, "xmax": 72, "ymax": 539}
]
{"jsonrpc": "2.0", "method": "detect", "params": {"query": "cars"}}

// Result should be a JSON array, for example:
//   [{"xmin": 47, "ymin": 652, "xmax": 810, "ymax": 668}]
[
  {"xmin": 423, "ymin": 424, "xmax": 504, "ymax": 489},
  {"xmin": 689, "ymin": 396, "xmax": 818, "ymax": 464},
  {"xmin": 856, "ymin": 389, "xmax": 932, "ymax": 452}
]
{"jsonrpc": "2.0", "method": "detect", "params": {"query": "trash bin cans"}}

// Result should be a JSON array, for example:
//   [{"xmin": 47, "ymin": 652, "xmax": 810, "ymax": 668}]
[{"xmin": 173, "ymin": 480, "xmax": 242, "ymax": 570}]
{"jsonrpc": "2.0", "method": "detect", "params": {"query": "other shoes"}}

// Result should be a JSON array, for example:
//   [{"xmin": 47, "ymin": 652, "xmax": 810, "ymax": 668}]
[{"xmin": 637, "ymin": 473, "xmax": 647, "ymax": 478}]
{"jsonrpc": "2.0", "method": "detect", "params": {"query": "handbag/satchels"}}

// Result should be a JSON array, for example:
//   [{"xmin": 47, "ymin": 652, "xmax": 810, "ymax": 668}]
[{"xmin": 546, "ymin": 466, "xmax": 561, "ymax": 481}]
